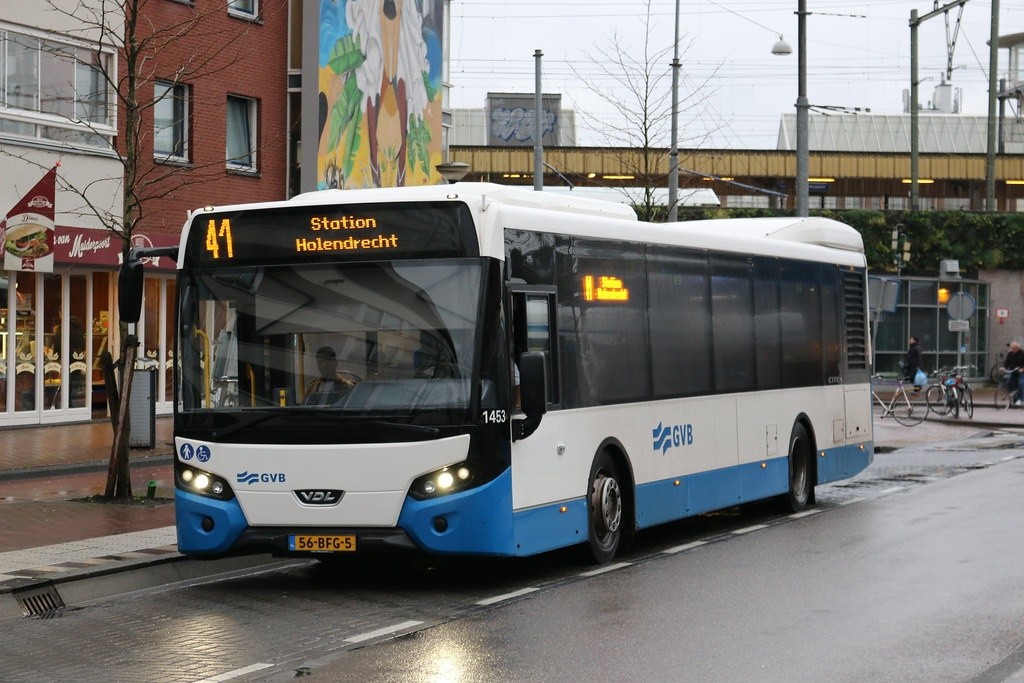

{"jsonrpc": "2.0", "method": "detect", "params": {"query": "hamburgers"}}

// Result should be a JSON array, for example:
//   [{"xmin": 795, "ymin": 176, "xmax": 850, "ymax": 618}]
[{"xmin": 6, "ymin": 224, "xmax": 48, "ymax": 256}]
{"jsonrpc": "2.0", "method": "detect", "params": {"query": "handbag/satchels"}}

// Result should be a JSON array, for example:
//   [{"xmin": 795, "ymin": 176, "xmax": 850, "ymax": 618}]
[{"xmin": 913, "ymin": 369, "xmax": 927, "ymax": 385}]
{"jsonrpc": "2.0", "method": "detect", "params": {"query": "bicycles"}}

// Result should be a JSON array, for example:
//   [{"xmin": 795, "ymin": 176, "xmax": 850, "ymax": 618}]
[
  {"xmin": 871, "ymin": 374, "xmax": 929, "ymax": 427},
  {"xmin": 925, "ymin": 365, "xmax": 979, "ymax": 420},
  {"xmin": 992, "ymin": 365, "xmax": 1024, "ymax": 413}
]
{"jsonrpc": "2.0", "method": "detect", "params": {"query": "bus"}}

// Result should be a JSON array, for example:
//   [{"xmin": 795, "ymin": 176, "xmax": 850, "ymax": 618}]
[{"xmin": 118, "ymin": 180, "xmax": 874, "ymax": 570}]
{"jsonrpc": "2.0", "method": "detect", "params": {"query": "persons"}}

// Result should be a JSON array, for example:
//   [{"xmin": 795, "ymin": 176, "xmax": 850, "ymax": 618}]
[
  {"xmin": 54, "ymin": 303, "xmax": 85, "ymax": 410},
  {"xmin": 907, "ymin": 335, "xmax": 924, "ymax": 396},
  {"xmin": 361, "ymin": 310, "xmax": 523, "ymax": 412},
  {"xmin": 999, "ymin": 341, "xmax": 1024, "ymax": 406},
  {"xmin": 307, "ymin": 346, "xmax": 354, "ymax": 409}
]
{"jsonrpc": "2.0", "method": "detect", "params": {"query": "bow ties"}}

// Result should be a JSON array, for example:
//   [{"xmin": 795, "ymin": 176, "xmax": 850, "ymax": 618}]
[{"xmin": 319, "ymin": 376, "xmax": 337, "ymax": 383}]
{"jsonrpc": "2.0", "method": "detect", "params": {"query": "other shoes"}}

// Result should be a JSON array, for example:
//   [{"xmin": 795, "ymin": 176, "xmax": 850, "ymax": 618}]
[
  {"xmin": 1015, "ymin": 400, "xmax": 1024, "ymax": 407},
  {"xmin": 911, "ymin": 391, "xmax": 920, "ymax": 396},
  {"xmin": 1009, "ymin": 390, "xmax": 1018, "ymax": 396}
]
